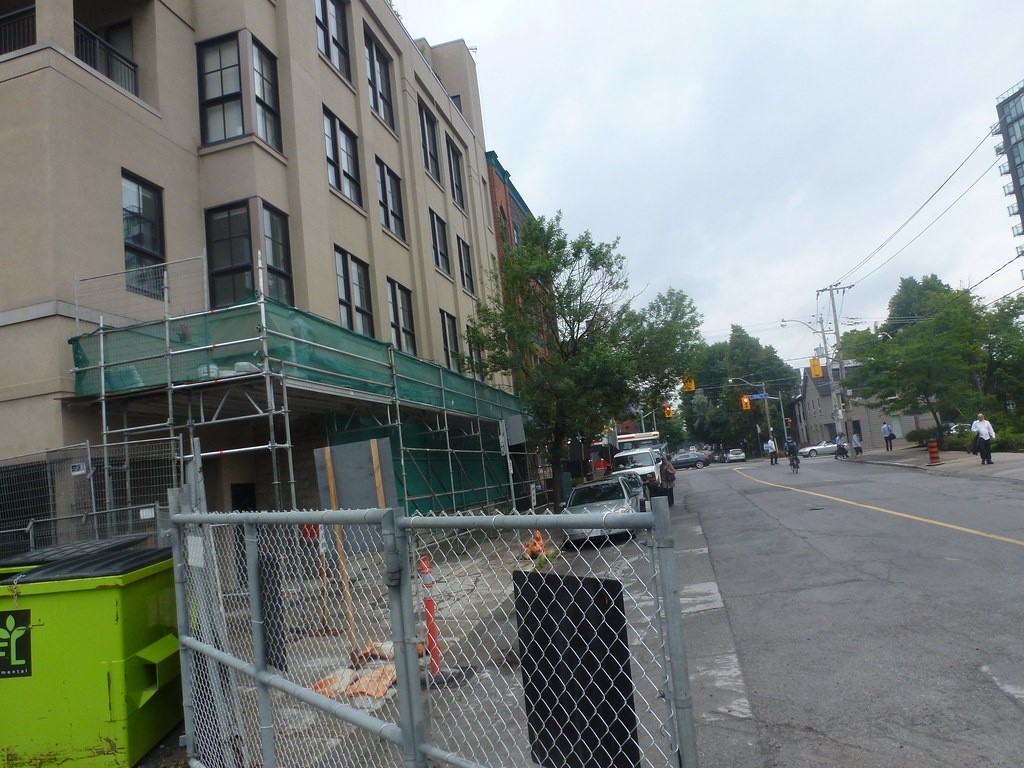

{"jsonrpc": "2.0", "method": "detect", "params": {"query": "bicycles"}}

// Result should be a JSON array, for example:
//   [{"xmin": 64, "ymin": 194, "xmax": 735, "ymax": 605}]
[{"xmin": 784, "ymin": 450, "xmax": 800, "ymax": 474}]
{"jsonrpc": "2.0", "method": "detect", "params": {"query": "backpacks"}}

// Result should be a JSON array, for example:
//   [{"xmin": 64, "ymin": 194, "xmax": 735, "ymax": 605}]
[{"xmin": 786, "ymin": 440, "xmax": 796, "ymax": 453}]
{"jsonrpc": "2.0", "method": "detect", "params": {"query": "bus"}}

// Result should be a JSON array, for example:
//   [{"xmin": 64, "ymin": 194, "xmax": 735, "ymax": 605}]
[{"xmin": 591, "ymin": 431, "xmax": 660, "ymax": 470}]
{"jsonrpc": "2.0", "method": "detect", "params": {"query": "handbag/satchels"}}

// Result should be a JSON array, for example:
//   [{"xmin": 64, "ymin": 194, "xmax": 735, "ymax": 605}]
[
  {"xmin": 889, "ymin": 434, "xmax": 895, "ymax": 439},
  {"xmin": 667, "ymin": 464, "xmax": 676, "ymax": 482}
]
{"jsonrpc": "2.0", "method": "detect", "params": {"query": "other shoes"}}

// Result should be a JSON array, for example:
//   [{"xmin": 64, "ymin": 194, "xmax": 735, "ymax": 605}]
[
  {"xmin": 982, "ymin": 459, "xmax": 985, "ymax": 464},
  {"xmin": 987, "ymin": 459, "xmax": 993, "ymax": 463}
]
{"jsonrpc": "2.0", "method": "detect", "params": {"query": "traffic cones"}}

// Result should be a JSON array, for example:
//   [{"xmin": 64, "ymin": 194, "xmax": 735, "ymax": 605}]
[{"xmin": 420, "ymin": 556, "xmax": 459, "ymax": 685}]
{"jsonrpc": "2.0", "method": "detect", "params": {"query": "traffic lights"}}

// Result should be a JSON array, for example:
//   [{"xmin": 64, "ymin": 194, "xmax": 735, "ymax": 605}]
[
  {"xmin": 742, "ymin": 397, "xmax": 750, "ymax": 410},
  {"xmin": 785, "ymin": 419, "xmax": 790, "ymax": 426},
  {"xmin": 665, "ymin": 404, "xmax": 672, "ymax": 417}
]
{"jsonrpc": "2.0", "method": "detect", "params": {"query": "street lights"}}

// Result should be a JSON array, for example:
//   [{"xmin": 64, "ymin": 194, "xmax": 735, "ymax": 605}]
[
  {"xmin": 781, "ymin": 318, "xmax": 842, "ymax": 434},
  {"xmin": 728, "ymin": 378, "xmax": 771, "ymax": 437}
]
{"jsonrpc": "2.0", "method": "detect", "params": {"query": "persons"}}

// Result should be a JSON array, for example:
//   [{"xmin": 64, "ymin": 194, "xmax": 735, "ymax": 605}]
[
  {"xmin": 287, "ymin": 310, "xmax": 319, "ymax": 376},
  {"xmin": 660, "ymin": 455, "xmax": 677, "ymax": 508},
  {"xmin": 785, "ymin": 436, "xmax": 800, "ymax": 468},
  {"xmin": 834, "ymin": 432, "xmax": 850, "ymax": 460},
  {"xmin": 852, "ymin": 431, "xmax": 866, "ymax": 461},
  {"xmin": 881, "ymin": 421, "xmax": 893, "ymax": 452},
  {"xmin": 767, "ymin": 435, "xmax": 779, "ymax": 465},
  {"xmin": 971, "ymin": 413, "xmax": 996, "ymax": 465}
]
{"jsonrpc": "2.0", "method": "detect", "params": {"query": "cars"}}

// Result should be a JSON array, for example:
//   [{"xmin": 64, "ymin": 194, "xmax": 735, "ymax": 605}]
[
  {"xmin": 798, "ymin": 440, "xmax": 849, "ymax": 458},
  {"xmin": 727, "ymin": 449, "xmax": 745, "ymax": 463},
  {"xmin": 670, "ymin": 453, "xmax": 710, "ymax": 469},
  {"xmin": 558, "ymin": 477, "xmax": 644, "ymax": 548},
  {"xmin": 945, "ymin": 424, "xmax": 972, "ymax": 434},
  {"xmin": 607, "ymin": 471, "xmax": 652, "ymax": 512}
]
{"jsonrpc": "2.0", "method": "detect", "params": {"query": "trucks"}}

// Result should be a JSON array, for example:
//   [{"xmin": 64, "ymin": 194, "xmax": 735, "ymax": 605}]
[{"xmin": 610, "ymin": 443, "xmax": 674, "ymax": 505}]
